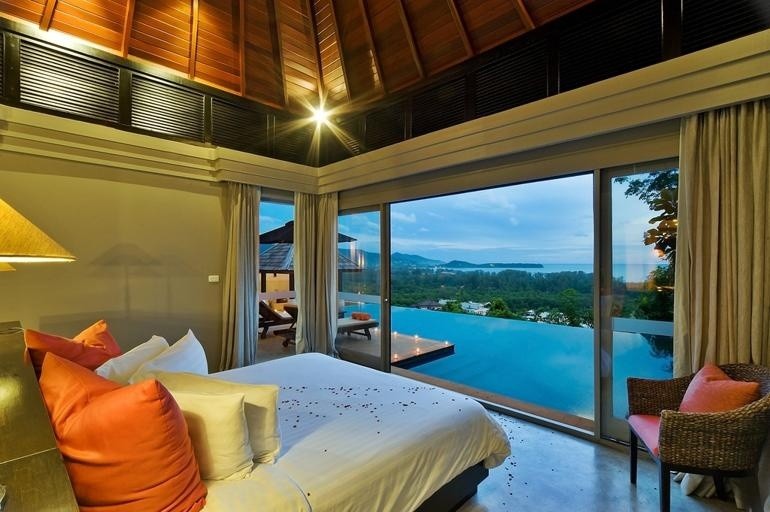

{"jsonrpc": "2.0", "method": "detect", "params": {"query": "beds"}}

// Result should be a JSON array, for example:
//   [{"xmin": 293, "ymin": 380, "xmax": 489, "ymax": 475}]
[{"xmin": 1, "ymin": 319, "xmax": 508, "ymax": 512}]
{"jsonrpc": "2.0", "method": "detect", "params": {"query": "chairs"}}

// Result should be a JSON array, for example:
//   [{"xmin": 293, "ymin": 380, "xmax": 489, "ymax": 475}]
[
  {"xmin": 624, "ymin": 361, "xmax": 769, "ymax": 512},
  {"xmin": 257, "ymin": 298, "xmax": 379, "ymax": 349}
]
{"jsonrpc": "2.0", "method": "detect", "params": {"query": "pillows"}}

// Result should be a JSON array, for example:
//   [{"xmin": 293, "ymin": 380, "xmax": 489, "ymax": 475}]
[
  {"xmin": 677, "ymin": 362, "xmax": 763, "ymax": 414},
  {"xmin": 23, "ymin": 318, "xmax": 121, "ymax": 381},
  {"xmin": 92, "ymin": 335, "xmax": 170, "ymax": 387},
  {"xmin": 140, "ymin": 368, "xmax": 256, "ymax": 482},
  {"xmin": 183, "ymin": 371, "xmax": 281, "ymax": 466},
  {"xmin": 127, "ymin": 328, "xmax": 210, "ymax": 384},
  {"xmin": 38, "ymin": 351, "xmax": 208, "ymax": 512}
]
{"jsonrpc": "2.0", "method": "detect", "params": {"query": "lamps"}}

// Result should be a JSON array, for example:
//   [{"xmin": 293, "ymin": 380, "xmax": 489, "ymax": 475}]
[{"xmin": 1, "ymin": 189, "xmax": 80, "ymax": 265}]
